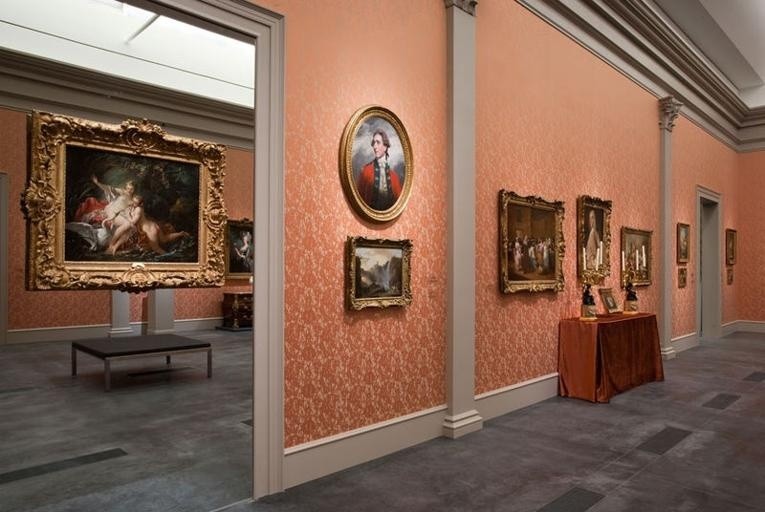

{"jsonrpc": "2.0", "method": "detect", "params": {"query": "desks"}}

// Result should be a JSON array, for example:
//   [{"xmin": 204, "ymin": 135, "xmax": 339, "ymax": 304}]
[
  {"xmin": 71, "ymin": 334, "xmax": 212, "ymax": 393},
  {"xmin": 557, "ymin": 311, "xmax": 664, "ymax": 404}
]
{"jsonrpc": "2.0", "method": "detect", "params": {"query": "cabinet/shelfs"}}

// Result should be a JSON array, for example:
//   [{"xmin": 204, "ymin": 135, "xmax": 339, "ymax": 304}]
[{"xmin": 222, "ymin": 292, "xmax": 254, "ymax": 330}]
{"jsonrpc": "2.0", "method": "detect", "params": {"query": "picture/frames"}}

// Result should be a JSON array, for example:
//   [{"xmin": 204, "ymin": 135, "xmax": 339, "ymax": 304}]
[
  {"xmin": 676, "ymin": 222, "xmax": 691, "ymax": 264},
  {"xmin": 577, "ymin": 195, "xmax": 613, "ymax": 279},
  {"xmin": 599, "ymin": 286, "xmax": 623, "ymax": 314},
  {"xmin": 224, "ymin": 217, "xmax": 255, "ymax": 281},
  {"xmin": 499, "ymin": 188, "xmax": 566, "ymax": 296},
  {"xmin": 338, "ymin": 104, "xmax": 414, "ymax": 224},
  {"xmin": 726, "ymin": 268, "xmax": 734, "ymax": 286},
  {"xmin": 19, "ymin": 108, "xmax": 229, "ymax": 293},
  {"xmin": 345, "ymin": 234, "xmax": 413, "ymax": 312},
  {"xmin": 620, "ymin": 225, "xmax": 654, "ymax": 290},
  {"xmin": 725, "ymin": 228, "xmax": 737, "ymax": 266},
  {"xmin": 677, "ymin": 267, "xmax": 688, "ymax": 288}
]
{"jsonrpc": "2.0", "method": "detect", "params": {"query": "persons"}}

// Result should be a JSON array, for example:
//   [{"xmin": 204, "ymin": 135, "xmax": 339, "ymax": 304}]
[
  {"xmin": 125, "ymin": 194, "xmax": 190, "ymax": 255},
  {"xmin": 89, "ymin": 175, "xmax": 137, "ymax": 257},
  {"xmin": 585, "ymin": 210, "xmax": 600, "ymax": 270},
  {"xmin": 507, "ymin": 235, "xmax": 553, "ymax": 274},
  {"xmin": 233, "ymin": 230, "xmax": 253, "ymax": 272},
  {"xmin": 358, "ymin": 128, "xmax": 402, "ymax": 211}
]
